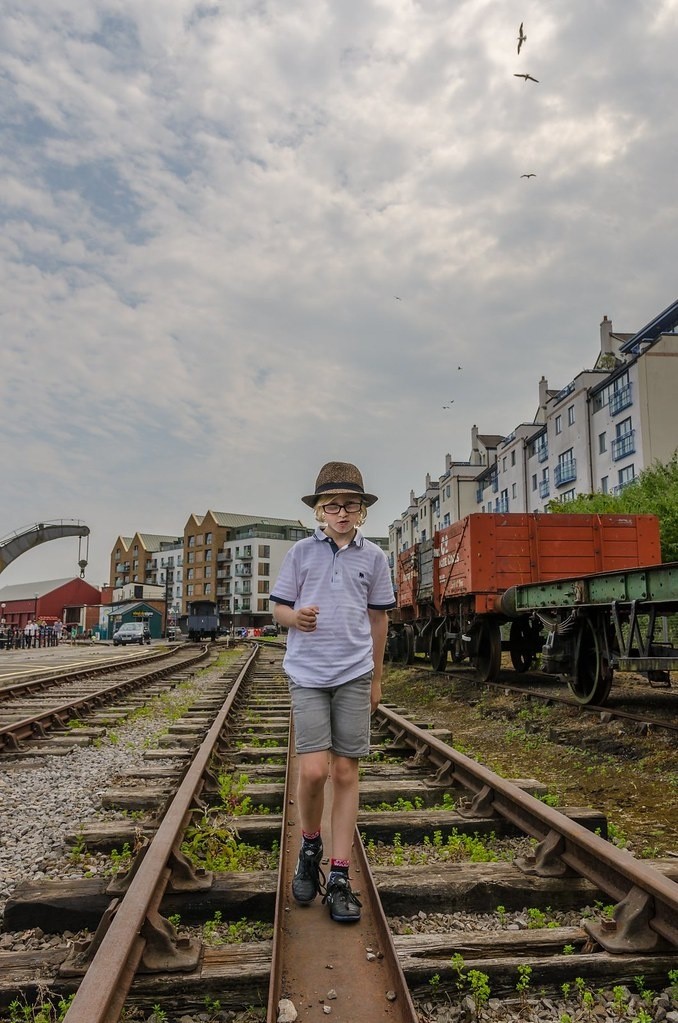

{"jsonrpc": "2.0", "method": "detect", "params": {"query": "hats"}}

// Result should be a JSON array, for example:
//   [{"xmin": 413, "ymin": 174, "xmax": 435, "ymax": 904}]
[{"xmin": 301, "ymin": 462, "xmax": 378, "ymax": 508}]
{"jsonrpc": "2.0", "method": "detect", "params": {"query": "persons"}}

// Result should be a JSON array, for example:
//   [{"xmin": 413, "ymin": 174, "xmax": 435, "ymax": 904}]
[
  {"xmin": 24, "ymin": 618, "xmax": 62, "ymax": 647},
  {"xmin": 268, "ymin": 462, "xmax": 397, "ymax": 924},
  {"xmin": 70, "ymin": 625, "xmax": 77, "ymax": 647}
]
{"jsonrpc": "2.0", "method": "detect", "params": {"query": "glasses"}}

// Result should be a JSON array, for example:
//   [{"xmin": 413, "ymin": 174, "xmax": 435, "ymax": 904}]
[{"xmin": 322, "ymin": 501, "xmax": 362, "ymax": 514}]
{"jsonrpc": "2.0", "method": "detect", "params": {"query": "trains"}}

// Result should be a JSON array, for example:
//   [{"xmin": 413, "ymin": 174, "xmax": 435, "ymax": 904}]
[
  {"xmin": 384, "ymin": 512, "xmax": 678, "ymax": 709},
  {"xmin": 186, "ymin": 598, "xmax": 220, "ymax": 643}
]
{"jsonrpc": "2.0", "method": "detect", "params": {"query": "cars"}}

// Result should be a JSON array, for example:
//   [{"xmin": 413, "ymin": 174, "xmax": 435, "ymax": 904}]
[
  {"xmin": 263, "ymin": 625, "xmax": 278, "ymax": 637},
  {"xmin": 220, "ymin": 626, "xmax": 263, "ymax": 638},
  {"xmin": 113, "ymin": 621, "xmax": 150, "ymax": 646}
]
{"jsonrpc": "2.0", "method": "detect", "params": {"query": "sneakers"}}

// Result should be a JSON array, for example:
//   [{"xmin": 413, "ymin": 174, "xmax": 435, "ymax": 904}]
[
  {"xmin": 321, "ymin": 871, "xmax": 362, "ymax": 920},
  {"xmin": 291, "ymin": 846, "xmax": 326, "ymax": 904}
]
